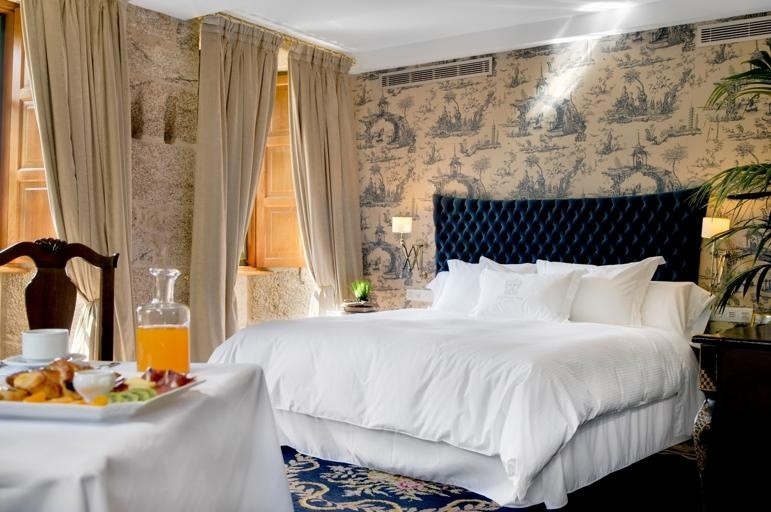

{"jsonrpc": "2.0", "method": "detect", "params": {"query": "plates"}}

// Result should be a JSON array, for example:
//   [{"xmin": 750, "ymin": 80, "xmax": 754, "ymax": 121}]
[
  {"xmin": 0, "ymin": 371, "xmax": 209, "ymax": 424},
  {"xmin": 1, "ymin": 354, "xmax": 87, "ymax": 367}
]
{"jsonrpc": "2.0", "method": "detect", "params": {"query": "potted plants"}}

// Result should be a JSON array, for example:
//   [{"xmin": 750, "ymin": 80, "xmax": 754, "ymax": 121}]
[{"xmin": 351, "ymin": 281, "xmax": 371, "ymax": 302}]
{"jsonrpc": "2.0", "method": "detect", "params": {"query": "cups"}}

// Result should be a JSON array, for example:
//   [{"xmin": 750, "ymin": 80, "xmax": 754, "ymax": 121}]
[{"xmin": 21, "ymin": 328, "xmax": 70, "ymax": 362}]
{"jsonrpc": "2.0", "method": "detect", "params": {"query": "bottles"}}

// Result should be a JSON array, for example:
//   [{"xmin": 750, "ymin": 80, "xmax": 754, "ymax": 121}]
[{"xmin": 134, "ymin": 266, "xmax": 191, "ymax": 375}]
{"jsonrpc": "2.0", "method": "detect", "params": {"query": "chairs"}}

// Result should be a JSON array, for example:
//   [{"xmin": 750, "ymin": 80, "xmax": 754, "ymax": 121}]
[{"xmin": 0, "ymin": 234, "xmax": 121, "ymax": 362}]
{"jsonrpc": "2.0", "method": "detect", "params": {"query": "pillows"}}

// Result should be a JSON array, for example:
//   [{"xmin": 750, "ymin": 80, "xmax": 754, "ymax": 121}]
[{"xmin": 425, "ymin": 254, "xmax": 717, "ymax": 344}]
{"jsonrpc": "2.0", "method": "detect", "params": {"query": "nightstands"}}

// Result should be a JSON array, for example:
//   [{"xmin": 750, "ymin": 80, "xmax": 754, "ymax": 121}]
[
  {"xmin": 689, "ymin": 321, "xmax": 771, "ymax": 511},
  {"xmin": 342, "ymin": 302, "xmax": 375, "ymax": 313}
]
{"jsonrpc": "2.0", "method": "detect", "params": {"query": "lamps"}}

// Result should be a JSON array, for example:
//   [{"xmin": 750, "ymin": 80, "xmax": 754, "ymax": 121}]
[
  {"xmin": 702, "ymin": 215, "xmax": 732, "ymax": 287},
  {"xmin": 391, "ymin": 216, "xmax": 426, "ymax": 279}
]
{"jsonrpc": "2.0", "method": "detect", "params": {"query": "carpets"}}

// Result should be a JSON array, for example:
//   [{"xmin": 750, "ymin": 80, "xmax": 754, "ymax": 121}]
[{"xmin": 282, "ymin": 450, "xmax": 536, "ymax": 512}]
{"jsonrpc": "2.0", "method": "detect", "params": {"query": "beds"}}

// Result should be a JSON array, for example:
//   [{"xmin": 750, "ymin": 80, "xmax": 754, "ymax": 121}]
[{"xmin": 215, "ymin": 185, "xmax": 717, "ymax": 512}]
